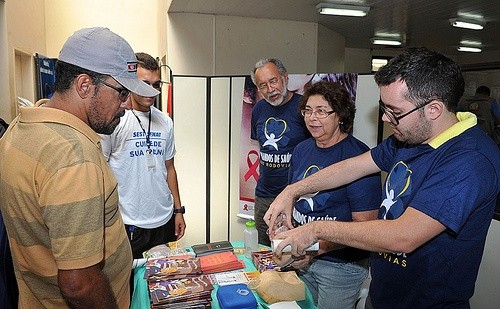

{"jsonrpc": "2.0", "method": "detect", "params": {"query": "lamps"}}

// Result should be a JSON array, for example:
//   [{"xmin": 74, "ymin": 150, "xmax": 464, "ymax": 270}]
[
  {"xmin": 315, "ymin": 2, "xmax": 370, "ymax": 16},
  {"xmin": 456, "ymin": 46, "xmax": 482, "ymax": 52},
  {"xmin": 450, "ymin": 18, "xmax": 483, "ymax": 29},
  {"xmin": 370, "ymin": 37, "xmax": 401, "ymax": 46}
]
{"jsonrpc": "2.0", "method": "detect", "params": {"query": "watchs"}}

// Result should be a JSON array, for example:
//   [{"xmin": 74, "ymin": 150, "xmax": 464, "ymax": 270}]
[{"xmin": 174, "ymin": 206, "xmax": 185, "ymax": 214}]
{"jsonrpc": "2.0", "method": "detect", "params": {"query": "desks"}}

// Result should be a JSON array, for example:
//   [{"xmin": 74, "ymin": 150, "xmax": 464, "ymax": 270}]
[{"xmin": 130, "ymin": 241, "xmax": 318, "ymax": 309}]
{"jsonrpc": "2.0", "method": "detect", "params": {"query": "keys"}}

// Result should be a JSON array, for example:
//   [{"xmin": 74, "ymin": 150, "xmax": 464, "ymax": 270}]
[{"xmin": 129, "ymin": 226, "xmax": 134, "ymax": 240}]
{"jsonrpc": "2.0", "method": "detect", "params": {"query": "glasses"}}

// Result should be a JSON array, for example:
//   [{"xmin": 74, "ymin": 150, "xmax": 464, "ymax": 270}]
[
  {"xmin": 258, "ymin": 74, "xmax": 282, "ymax": 92},
  {"xmin": 142, "ymin": 80, "xmax": 164, "ymax": 91},
  {"xmin": 96, "ymin": 78, "xmax": 131, "ymax": 102},
  {"xmin": 301, "ymin": 109, "xmax": 336, "ymax": 119},
  {"xmin": 379, "ymin": 98, "xmax": 448, "ymax": 125}
]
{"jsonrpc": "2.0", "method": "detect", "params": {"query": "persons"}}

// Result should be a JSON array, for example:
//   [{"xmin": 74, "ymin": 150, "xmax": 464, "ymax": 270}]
[
  {"xmin": 0, "ymin": 28, "xmax": 162, "ymax": 309},
  {"xmin": 287, "ymin": 81, "xmax": 381, "ymax": 309},
  {"xmin": 96, "ymin": 52, "xmax": 187, "ymax": 309},
  {"xmin": 474, "ymin": 85, "xmax": 500, "ymax": 118},
  {"xmin": 263, "ymin": 47, "xmax": 500, "ymax": 309},
  {"xmin": 249, "ymin": 59, "xmax": 313, "ymax": 249}
]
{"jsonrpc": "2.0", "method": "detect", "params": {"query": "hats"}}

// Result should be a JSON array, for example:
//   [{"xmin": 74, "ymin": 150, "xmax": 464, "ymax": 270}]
[{"xmin": 57, "ymin": 28, "xmax": 161, "ymax": 97}]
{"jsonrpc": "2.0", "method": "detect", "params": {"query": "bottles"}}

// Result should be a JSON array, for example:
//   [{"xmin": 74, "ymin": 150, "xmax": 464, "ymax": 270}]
[{"xmin": 244, "ymin": 217, "xmax": 259, "ymax": 258}]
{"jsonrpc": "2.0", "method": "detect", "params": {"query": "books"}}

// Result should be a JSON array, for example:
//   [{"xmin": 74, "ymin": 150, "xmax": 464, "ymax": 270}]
[
  {"xmin": 251, "ymin": 251, "xmax": 300, "ymax": 277},
  {"xmin": 143, "ymin": 257, "xmax": 215, "ymax": 309}
]
